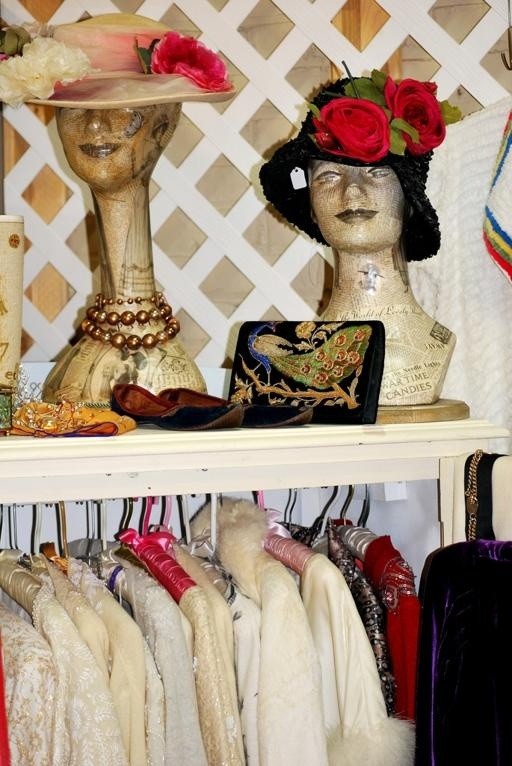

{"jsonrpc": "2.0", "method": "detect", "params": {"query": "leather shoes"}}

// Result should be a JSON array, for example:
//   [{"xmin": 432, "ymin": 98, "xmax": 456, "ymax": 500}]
[{"xmin": 112, "ymin": 384, "xmax": 313, "ymax": 431}]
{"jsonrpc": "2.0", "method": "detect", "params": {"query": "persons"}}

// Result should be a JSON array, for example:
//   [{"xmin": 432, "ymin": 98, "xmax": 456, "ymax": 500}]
[
  {"xmin": 258, "ymin": 78, "xmax": 455, "ymax": 411},
  {"xmin": 1, "ymin": 10, "xmax": 243, "ymax": 411}
]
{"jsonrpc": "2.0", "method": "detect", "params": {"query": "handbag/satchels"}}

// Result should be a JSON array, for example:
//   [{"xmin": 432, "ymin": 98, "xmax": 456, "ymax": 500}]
[{"xmin": 229, "ymin": 321, "xmax": 386, "ymax": 425}]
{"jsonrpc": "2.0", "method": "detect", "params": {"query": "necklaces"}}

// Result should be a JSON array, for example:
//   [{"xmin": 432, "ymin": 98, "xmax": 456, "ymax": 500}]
[{"xmin": 78, "ymin": 288, "xmax": 181, "ymax": 353}]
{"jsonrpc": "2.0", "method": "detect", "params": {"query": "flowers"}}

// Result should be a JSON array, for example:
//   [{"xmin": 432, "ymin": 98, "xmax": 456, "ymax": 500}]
[
  {"xmin": 0, "ymin": 22, "xmax": 99, "ymax": 105},
  {"xmin": 304, "ymin": 56, "xmax": 462, "ymax": 164},
  {"xmin": 133, "ymin": 31, "xmax": 235, "ymax": 94}
]
